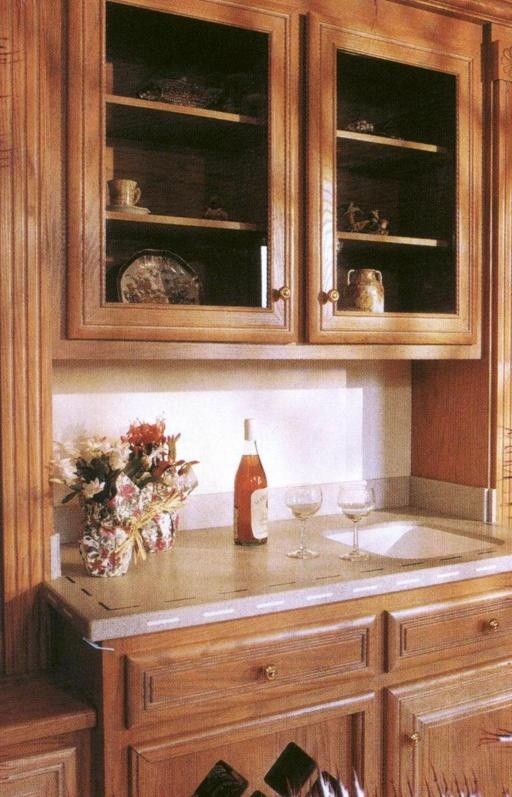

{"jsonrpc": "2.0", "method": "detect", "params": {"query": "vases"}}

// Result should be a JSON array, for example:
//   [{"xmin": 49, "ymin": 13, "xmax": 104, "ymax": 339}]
[
  {"xmin": 79, "ymin": 527, "xmax": 134, "ymax": 576},
  {"xmin": 137, "ymin": 513, "xmax": 177, "ymax": 555}
]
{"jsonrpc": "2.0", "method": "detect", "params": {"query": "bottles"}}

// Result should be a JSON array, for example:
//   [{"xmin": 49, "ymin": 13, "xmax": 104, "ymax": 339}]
[
  {"xmin": 345, "ymin": 270, "xmax": 387, "ymax": 314},
  {"xmin": 235, "ymin": 419, "xmax": 269, "ymax": 548}
]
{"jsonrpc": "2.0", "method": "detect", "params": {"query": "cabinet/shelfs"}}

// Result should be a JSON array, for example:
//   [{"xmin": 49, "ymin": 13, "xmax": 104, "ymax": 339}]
[
  {"xmin": 66, "ymin": 0, "xmax": 483, "ymax": 360},
  {"xmin": 0, "ymin": 666, "xmax": 100, "ymax": 797},
  {"xmin": 55, "ymin": 575, "xmax": 512, "ymax": 797}
]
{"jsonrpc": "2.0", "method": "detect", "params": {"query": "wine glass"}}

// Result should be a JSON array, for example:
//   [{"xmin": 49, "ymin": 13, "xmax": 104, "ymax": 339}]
[
  {"xmin": 285, "ymin": 485, "xmax": 322, "ymax": 563},
  {"xmin": 335, "ymin": 480, "xmax": 376, "ymax": 564}
]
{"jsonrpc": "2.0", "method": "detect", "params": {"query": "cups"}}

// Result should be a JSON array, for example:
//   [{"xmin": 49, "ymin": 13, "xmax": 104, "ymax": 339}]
[{"xmin": 107, "ymin": 176, "xmax": 139, "ymax": 203}]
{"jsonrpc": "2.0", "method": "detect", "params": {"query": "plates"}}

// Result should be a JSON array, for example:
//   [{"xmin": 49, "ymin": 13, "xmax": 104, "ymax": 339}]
[
  {"xmin": 115, "ymin": 249, "xmax": 204, "ymax": 305},
  {"xmin": 109, "ymin": 203, "xmax": 152, "ymax": 215}
]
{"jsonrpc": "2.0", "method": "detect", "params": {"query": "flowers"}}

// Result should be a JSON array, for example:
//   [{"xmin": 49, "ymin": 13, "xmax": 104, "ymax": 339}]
[
  {"xmin": 49, "ymin": 418, "xmax": 181, "ymax": 563},
  {"xmin": 119, "ymin": 421, "xmax": 196, "ymax": 512}
]
